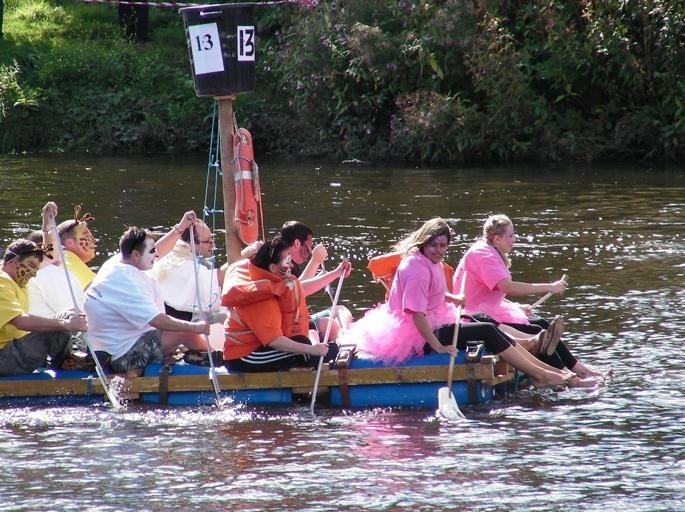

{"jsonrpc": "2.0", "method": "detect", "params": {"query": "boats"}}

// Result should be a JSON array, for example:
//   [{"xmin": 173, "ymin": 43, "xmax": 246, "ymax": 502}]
[{"xmin": 0, "ymin": 0, "xmax": 566, "ymax": 412}]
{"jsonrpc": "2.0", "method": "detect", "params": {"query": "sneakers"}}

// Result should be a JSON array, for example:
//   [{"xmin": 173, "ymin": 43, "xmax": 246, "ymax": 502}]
[{"xmin": 529, "ymin": 314, "xmax": 564, "ymax": 357}]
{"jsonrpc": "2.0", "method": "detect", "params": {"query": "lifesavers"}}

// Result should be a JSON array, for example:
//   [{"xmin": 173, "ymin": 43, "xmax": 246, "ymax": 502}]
[{"xmin": 233, "ymin": 127, "xmax": 258, "ymax": 245}]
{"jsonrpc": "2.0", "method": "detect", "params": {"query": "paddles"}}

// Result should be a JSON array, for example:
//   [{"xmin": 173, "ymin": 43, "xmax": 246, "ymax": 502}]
[
  {"xmin": 188, "ymin": 215, "xmax": 223, "ymax": 410},
  {"xmin": 51, "ymin": 214, "xmax": 123, "ymax": 409},
  {"xmin": 438, "ymin": 270, "xmax": 465, "ymax": 422}
]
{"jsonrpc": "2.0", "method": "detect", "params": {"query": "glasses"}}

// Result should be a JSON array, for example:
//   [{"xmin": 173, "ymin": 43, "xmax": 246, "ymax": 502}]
[{"xmin": 195, "ymin": 238, "xmax": 214, "ymax": 244}]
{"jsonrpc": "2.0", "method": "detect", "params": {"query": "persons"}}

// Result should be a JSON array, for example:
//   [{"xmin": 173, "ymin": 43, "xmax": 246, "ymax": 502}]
[
  {"xmin": 452, "ymin": 213, "xmax": 615, "ymax": 390},
  {"xmin": 382, "ymin": 216, "xmax": 579, "ymax": 389},
  {"xmin": 369, "ymin": 232, "xmax": 566, "ymax": 359},
  {"xmin": 0, "ymin": 201, "xmax": 97, "ymax": 378},
  {"xmin": 84, "ymin": 211, "xmax": 225, "ymax": 376},
  {"xmin": 221, "ymin": 221, "xmax": 353, "ymax": 373}
]
